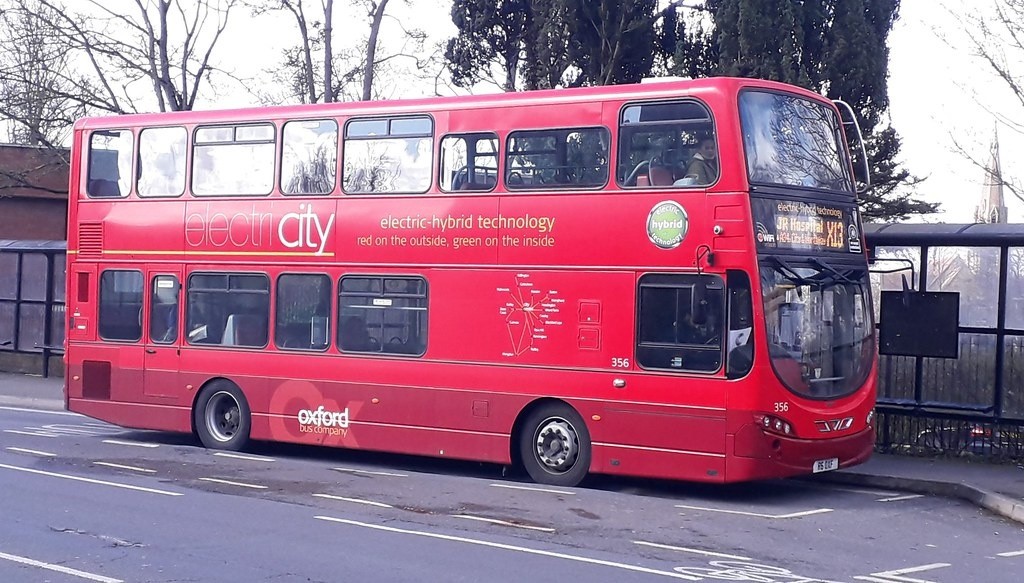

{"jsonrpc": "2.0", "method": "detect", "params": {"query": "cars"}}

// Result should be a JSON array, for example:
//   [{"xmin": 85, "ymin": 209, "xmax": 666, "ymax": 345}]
[{"xmin": 917, "ymin": 425, "xmax": 1024, "ymax": 456}]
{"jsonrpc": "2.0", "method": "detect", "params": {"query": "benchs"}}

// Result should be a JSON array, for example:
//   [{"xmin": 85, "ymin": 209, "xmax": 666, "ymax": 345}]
[
  {"xmin": 220, "ymin": 313, "xmax": 265, "ymax": 345},
  {"xmin": 138, "ymin": 303, "xmax": 172, "ymax": 340},
  {"xmin": 877, "ymin": 398, "xmax": 993, "ymax": 448},
  {"xmin": 34, "ymin": 345, "xmax": 65, "ymax": 351}
]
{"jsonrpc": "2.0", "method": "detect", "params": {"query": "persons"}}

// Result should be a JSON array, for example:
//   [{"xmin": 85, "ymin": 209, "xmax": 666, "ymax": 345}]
[
  {"xmin": 166, "ymin": 282, "xmax": 208, "ymax": 342},
  {"xmin": 685, "ymin": 135, "xmax": 720, "ymax": 185}
]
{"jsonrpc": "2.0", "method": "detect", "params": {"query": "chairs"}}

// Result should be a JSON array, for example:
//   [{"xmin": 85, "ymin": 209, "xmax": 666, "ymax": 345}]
[
  {"xmin": 282, "ymin": 337, "xmax": 301, "ymax": 348},
  {"xmin": 507, "ymin": 172, "xmax": 524, "ymax": 184},
  {"xmin": 387, "ymin": 337, "xmax": 403, "ymax": 352},
  {"xmin": 369, "ymin": 337, "xmax": 380, "ymax": 350},
  {"xmin": 340, "ymin": 316, "xmax": 370, "ymax": 351},
  {"xmin": 648, "ymin": 155, "xmax": 675, "ymax": 186}
]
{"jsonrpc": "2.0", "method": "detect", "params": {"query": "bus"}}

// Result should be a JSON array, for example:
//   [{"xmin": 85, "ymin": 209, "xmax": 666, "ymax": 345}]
[{"xmin": 62, "ymin": 77, "xmax": 915, "ymax": 487}]
